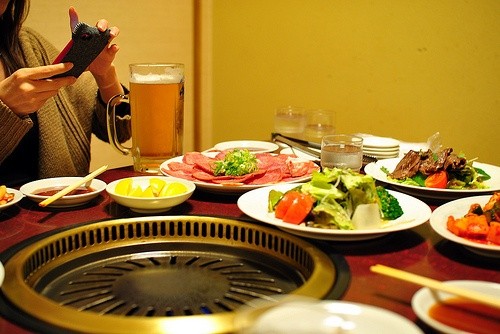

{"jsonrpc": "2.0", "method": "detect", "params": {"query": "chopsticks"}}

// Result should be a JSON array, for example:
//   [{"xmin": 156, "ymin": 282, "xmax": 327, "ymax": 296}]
[
  {"xmin": 370, "ymin": 264, "xmax": 500, "ymax": 310},
  {"xmin": 38, "ymin": 164, "xmax": 109, "ymax": 207}
]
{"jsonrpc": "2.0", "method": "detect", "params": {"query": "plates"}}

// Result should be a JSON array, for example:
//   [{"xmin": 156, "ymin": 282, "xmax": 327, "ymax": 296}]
[
  {"xmin": 249, "ymin": 298, "xmax": 424, "ymax": 334},
  {"xmin": 236, "ymin": 182, "xmax": 433, "ymax": 242},
  {"xmin": 214, "ymin": 140, "xmax": 279, "ymax": 155},
  {"xmin": 412, "ymin": 279, "xmax": 500, "ymax": 334},
  {"xmin": 360, "ymin": 135, "xmax": 399, "ymax": 159},
  {"xmin": 363, "ymin": 156, "xmax": 500, "ymax": 200},
  {"xmin": 105, "ymin": 175, "xmax": 196, "ymax": 214},
  {"xmin": 429, "ymin": 195, "xmax": 500, "ymax": 258},
  {"xmin": 159, "ymin": 151, "xmax": 321, "ymax": 195},
  {"xmin": 0, "ymin": 187, "xmax": 23, "ymax": 208},
  {"xmin": 19, "ymin": 177, "xmax": 108, "ymax": 207}
]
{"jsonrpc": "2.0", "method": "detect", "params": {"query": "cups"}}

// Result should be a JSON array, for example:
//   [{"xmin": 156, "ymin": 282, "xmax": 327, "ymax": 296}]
[
  {"xmin": 319, "ymin": 134, "xmax": 364, "ymax": 175},
  {"xmin": 106, "ymin": 62, "xmax": 185, "ymax": 175},
  {"xmin": 275, "ymin": 104, "xmax": 305, "ymax": 139},
  {"xmin": 303, "ymin": 107, "xmax": 336, "ymax": 147}
]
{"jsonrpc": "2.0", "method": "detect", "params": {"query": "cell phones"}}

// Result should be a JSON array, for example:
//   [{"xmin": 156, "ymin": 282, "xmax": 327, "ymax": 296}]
[{"xmin": 47, "ymin": 22, "xmax": 111, "ymax": 78}]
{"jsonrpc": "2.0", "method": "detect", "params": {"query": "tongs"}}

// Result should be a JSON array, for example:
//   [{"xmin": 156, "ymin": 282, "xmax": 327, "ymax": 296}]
[{"xmin": 271, "ymin": 132, "xmax": 379, "ymax": 173}]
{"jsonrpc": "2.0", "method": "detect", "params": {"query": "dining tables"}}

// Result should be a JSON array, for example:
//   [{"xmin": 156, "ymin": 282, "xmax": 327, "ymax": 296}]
[{"xmin": 0, "ymin": 165, "xmax": 500, "ymax": 334}]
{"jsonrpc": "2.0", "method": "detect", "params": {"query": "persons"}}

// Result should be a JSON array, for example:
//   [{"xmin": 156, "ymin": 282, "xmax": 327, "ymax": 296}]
[{"xmin": 0, "ymin": 0, "xmax": 132, "ymax": 188}]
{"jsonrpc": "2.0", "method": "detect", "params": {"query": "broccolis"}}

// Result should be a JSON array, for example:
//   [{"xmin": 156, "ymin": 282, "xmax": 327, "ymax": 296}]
[{"xmin": 375, "ymin": 188, "xmax": 404, "ymax": 219}]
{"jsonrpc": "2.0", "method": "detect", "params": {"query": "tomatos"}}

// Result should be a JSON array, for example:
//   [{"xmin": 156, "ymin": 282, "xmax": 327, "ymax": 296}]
[
  {"xmin": 284, "ymin": 193, "xmax": 315, "ymax": 224},
  {"xmin": 274, "ymin": 190, "xmax": 300, "ymax": 220},
  {"xmin": 425, "ymin": 171, "xmax": 447, "ymax": 189}
]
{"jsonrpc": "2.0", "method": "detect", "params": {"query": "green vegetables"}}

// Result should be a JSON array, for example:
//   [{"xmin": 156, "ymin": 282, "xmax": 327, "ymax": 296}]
[
  {"xmin": 380, "ymin": 151, "xmax": 490, "ymax": 190},
  {"xmin": 213, "ymin": 148, "xmax": 259, "ymax": 176},
  {"xmin": 269, "ymin": 166, "xmax": 383, "ymax": 230}
]
